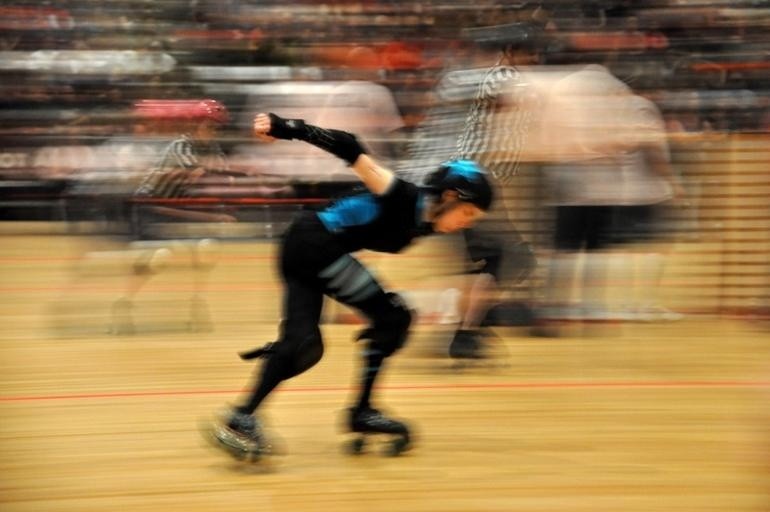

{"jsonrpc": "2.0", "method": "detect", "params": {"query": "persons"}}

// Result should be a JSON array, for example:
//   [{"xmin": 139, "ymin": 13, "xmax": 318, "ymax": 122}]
[
  {"xmin": 207, "ymin": 110, "xmax": 496, "ymax": 461},
  {"xmin": 550, "ymin": 35, "xmax": 636, "ymax": 328},
  {"xmin": 438, "ymin": 24, "xmax": 541, "ymax": 357},
  {"xmin": 0, "ymin": 0, "xmax": 768, "ymax": 358}
]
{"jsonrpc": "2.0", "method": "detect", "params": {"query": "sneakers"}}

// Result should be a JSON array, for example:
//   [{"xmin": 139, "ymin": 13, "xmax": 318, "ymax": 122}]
[{"xmin": 348, "ymin": 404, "xmax": 411, "ymax": 436}]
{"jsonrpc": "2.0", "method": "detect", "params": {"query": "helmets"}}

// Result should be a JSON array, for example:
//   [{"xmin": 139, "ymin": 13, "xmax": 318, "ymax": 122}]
[
  {"xmin": 423, "ymin": 158, "xmax": 496, "ymax": 213},
  {"xmin": 196, "ymin": 96, "xmax": 230, "ymax": 119}
]
